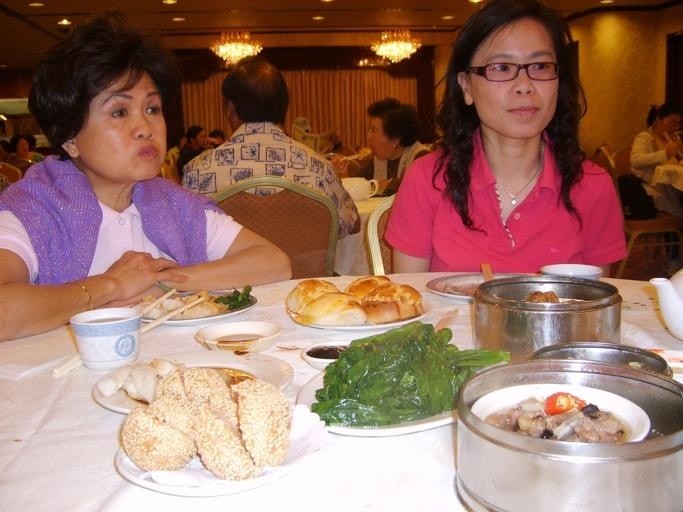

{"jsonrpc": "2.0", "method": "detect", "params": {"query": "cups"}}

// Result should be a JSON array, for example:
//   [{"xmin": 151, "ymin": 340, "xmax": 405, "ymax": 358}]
[
  {"xmin": 340, "ymin": 177, "xmax": 379, "ymax": 202},
  {"xmin": 66, "ymin": 306, "xmax": 141, "ymax": 372}
]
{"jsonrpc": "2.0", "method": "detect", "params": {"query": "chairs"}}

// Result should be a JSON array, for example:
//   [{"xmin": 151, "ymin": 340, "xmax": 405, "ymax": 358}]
[
  {"xmin": 197, "ymin": 175, "xmax": 342, "ymax": 278},
  {"xmin": 594, "ymin": 142, "xmax": 681, "ymax": 282},
  {"xmin": 364, "ymin": 194, "xmax": 401, "ymax": 271},
  {"xmin": 1, "ymin": 142, "xmax": 44, "ymax": 186}
]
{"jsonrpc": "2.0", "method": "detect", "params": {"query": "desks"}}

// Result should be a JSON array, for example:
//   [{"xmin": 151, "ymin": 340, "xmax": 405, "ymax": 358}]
[{"xmin": 343, "ymin": 192, "xmax": 390, "ymax": 271}]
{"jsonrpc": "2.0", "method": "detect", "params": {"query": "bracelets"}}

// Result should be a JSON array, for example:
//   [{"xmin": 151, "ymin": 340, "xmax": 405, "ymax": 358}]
[
  {"xmin": 76, "ymin": 280, "xmax": 93, "ymax": 311},
  {"xmin": 153, "ymin": 282, "xmax": 172, "ymax": 293}
]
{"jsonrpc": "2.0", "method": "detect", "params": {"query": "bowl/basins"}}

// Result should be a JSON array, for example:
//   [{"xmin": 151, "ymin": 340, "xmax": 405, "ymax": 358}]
[
  {"xmin": 538, "ymin": 264, "xmax": 602, "ymax": 282},
  {"xmin": 468, "ymin": 381, "xmax": 652, "ymax": 444},
  {"xmin": 530, "ymin": 341, "xmax": 673, "ymax": 380}
]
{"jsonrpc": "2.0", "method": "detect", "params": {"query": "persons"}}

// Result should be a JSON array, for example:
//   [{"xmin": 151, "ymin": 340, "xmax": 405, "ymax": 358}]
[
  {"xmin": 180, "ymin": 55, "xmax": 361, "ymax": 238},
  {"xmin": 631, "ymin": 102, "xmax": 683, "ymax": 257},
  {"xmin": 167, "ymin": 125, "xmax": 224, "ymax": 175},
  {"xmin": 383, "ymin": 0, "xmax": 630, "ymax": 277},
  {"xmin": 0, "ymin": 11, "xmax": 292, "ymax": 342},
  {"xmin": 331, "ymin": 98, "xmax": 432, "ymax": 195},
  {"xmin": 0, "ymin": 133, "xmax": 46, "ymax": 189}
]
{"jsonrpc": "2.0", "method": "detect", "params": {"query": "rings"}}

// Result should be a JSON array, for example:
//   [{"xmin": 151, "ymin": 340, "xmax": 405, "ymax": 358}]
[{"xmin": 340, "ymin": 166, "xmax": 343, "ymax": 170}]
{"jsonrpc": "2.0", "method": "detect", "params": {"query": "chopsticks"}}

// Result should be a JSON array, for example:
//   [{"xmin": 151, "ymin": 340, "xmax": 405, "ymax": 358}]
[
  {"xmin": 662, "ymin": 132, "xmax": 683, "ymax": 158},
  {"xmin": 328, "ymin": 153, "xmax": 361, "ymax": 165},
  {"xmin": 479, "ymin": 263, "xmax": 495, "ymax": 282},
  {"xmin": 52, "ymin": 288, "xmax": 206, "ymax": 379}
]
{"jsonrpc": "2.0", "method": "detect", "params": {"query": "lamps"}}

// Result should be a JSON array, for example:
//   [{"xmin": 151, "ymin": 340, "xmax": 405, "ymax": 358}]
[
  {"xmin": 208, "ymin": 30, "xmax": 265, "ymax": 68},
  {"xmin": 367, "ymin": 27, "xmax": 423, "ymax": 65}
]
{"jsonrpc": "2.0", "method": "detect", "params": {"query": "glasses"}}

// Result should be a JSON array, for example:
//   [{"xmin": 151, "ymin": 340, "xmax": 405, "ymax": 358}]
[{"xmin": 468, "ymin": 61, "xmax": 560, "ymax": 81}]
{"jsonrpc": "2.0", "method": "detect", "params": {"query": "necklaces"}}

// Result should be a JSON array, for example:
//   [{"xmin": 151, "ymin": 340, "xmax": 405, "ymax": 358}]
[{"xmin": 494, "ymin": 161, "xmax": 543, "ymax": 204}]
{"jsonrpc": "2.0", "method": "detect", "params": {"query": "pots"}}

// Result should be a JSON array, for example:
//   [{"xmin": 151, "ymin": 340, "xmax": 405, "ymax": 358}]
[{"xmin": 473, "ymin": 275, "xmax": 622, "ymax": 357}]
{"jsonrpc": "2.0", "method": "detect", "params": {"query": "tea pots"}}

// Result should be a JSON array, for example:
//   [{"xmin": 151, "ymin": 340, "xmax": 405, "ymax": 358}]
[{"xmin": 650, "ymin": 269, "xmax": 683, "ymax": 341}]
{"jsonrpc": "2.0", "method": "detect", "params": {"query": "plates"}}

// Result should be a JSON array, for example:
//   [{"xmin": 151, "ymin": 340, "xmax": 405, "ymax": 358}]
[
  {"xmin": 92, "ymin": 350, "xmax": 293, "ymax": 415},
  {"xmin": 193, "ymin": 322, "xmax": 282, "ymax": 353},
  {"xmin": 115, "ymin": 443, "xmax": 271, "ymax": 497},
  {"xmin": 424, "ymin": 270, "xmax": 526, "ymax": 304},
  {"xmin": 301, "ymin": 343, "xmax": 345, "ymax": 370},
  {"xmin": 295, "ymin": 362, "xmax": 508, "ymax": 438},
  {"xmin": 286, "ymin": 298, "xmax": 433, "ymax": 332},
  {"xmin": 136, "ymin": 289, "xmax": 258, "ymax": 328}
]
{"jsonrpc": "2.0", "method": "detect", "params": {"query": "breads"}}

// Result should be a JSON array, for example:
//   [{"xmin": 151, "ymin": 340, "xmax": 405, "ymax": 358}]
[
  {"xmin": 121, "ymin": 367, "xmax": 290, "ymax": 481},
  {"xmin": 285, "ymin": 275, "xmax": 424, "ymax": 326}
]
